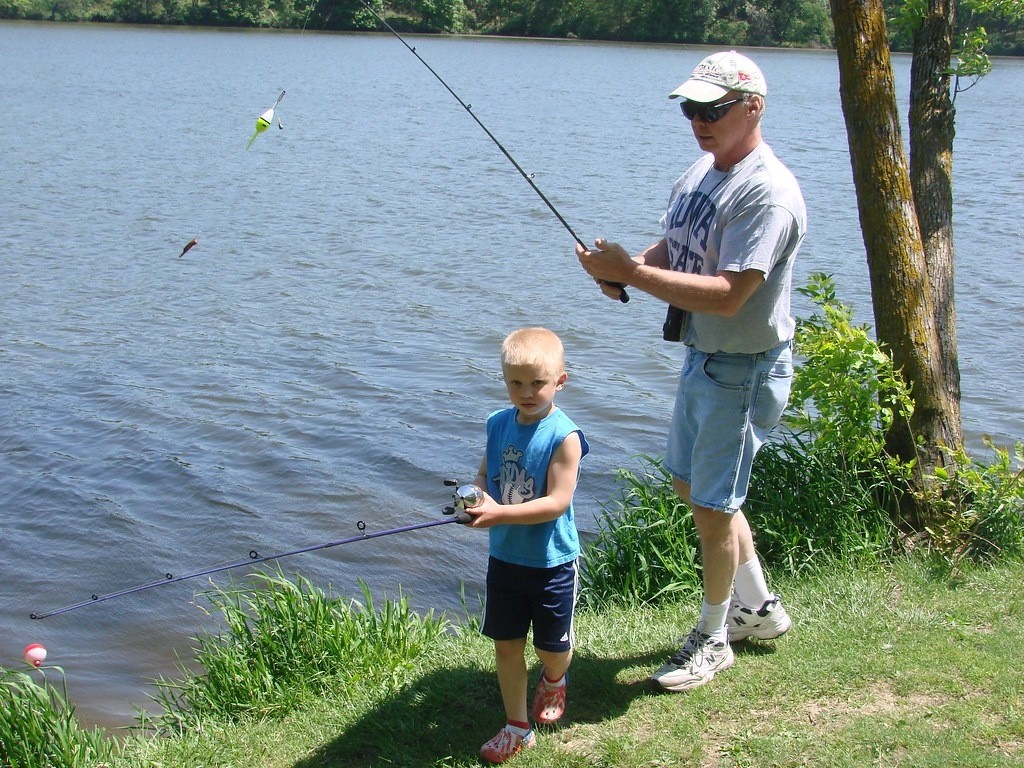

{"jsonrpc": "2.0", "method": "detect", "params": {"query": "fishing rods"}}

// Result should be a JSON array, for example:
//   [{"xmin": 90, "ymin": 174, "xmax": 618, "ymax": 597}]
[
  {"xmin": 30, "ymin": 479, "xmax": 481, "ymax": 620},
  {"xmin": 359, "ymin": 0, "xmax": 630, "ymax": 303}
]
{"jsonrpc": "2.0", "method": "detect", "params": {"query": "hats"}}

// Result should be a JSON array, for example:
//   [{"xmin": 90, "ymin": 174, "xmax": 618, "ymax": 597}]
[{"xmin": 668, "ymin": 50, "xmax": 767, "ymax": 102}]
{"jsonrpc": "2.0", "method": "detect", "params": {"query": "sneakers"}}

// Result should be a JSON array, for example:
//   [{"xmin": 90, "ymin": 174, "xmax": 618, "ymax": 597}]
[
  {"xmin": 650, "ymin": 623, "xmax": 734, "ymax": 691},
  {"xmin": 726, "ymin": 590, "xmax": 792, "ymax": 641}
]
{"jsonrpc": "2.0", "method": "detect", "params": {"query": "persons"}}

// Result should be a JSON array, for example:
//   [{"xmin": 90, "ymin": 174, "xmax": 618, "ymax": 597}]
[
  {"xmin": 575, "ymin": 50, "xmax": 808, "ymax": 691},
  {"xmin": 471, "ymin": 327, "xmax": 590, "ymax": 765}
]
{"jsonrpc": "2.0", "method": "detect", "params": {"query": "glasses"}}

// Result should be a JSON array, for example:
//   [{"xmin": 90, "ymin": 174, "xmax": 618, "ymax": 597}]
[{"xmin": 680, "ymin": 96, "xmax": 750, "ymax": 122}]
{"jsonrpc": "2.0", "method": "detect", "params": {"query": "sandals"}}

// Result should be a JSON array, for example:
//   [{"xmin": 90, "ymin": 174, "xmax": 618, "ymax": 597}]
[
  {"xmin": 480, "ymin": 725, "xmax": 537, "ymax": 763},
  {"xmin": 534, "ymin": 667, "xmax": 567, "ymax": 723}
]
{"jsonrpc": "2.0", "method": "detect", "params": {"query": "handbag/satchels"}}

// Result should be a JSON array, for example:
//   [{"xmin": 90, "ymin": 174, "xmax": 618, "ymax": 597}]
[{"xmin": 663, "ymin": 303, "xmax": 690, "ymax": 341}]
